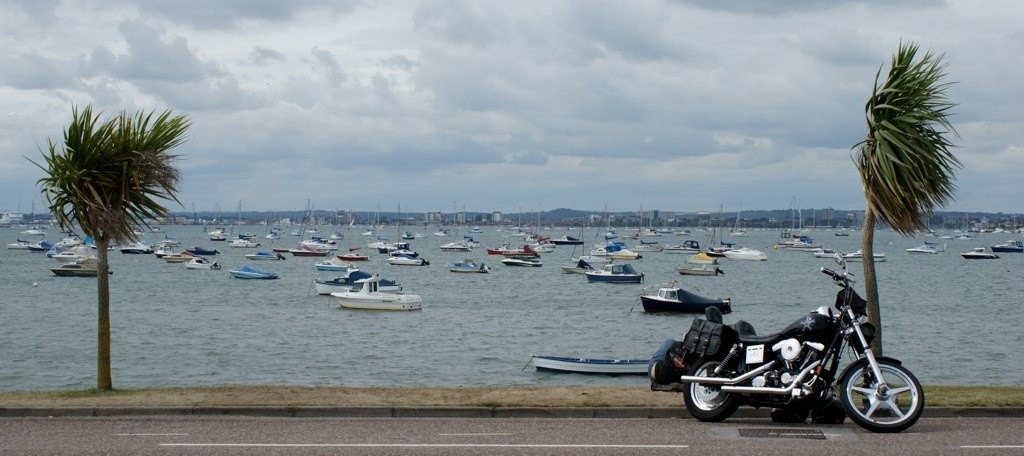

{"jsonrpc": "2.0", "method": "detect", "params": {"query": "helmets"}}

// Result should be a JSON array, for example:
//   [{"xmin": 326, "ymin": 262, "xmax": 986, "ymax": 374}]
[
  {"xmin": 770, "ymin": 396, "xmax": 811, "ymax": 422},
  {"xmin": 811, "ymin": 395, "xmax": 846, "ymax": 424}
]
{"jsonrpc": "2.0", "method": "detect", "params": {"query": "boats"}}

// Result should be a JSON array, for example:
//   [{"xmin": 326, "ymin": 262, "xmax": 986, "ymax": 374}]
[
  {"xmin": 990, "ymin": 239, "xmax": 1024, "ymax": 253},
  {"xmin": 959, "ymin": 247, "xmax": 1002, "ymax": 259},
  {"xmin": 499, "ymin": 251, "xmax": 544, "ymax": 268},
  {"xmin": 182, "ymin": 256, "xmax": 222, "ymax": 271},
  {"xmin": 329, "ymin": 270, "xmax": 423, "ymax": 311},
  {"xmin": 314, "ymin": 252, "xmax": 350, "ymax": 273},
  {"xmin": 447, "ymin": 256, "xmax": 492, "ymax": 274},
  {"xmin": 529, "ymin": 352, "xmax": 650, "ymax": 375},
  {"xmin": 639, "ymin": 278, "xmax": 732, "ymax": 315},
  {"xmin": 48, "ymin": 256, "xmax": 114, "ymax": 277},
  {"xmin": 310, "ymin": 261, "xmax": 403, "ymax": 296},
  {"xmin": 227, "ymin": 262, "xmax": 279, "ymax": 280},
  {"xmin": 673, "ymin": 256, "xmax": 725, "ymax": 277},
  {"xmin": 583, "ymin": 263, "xmax": 645, "ymax": 284}
]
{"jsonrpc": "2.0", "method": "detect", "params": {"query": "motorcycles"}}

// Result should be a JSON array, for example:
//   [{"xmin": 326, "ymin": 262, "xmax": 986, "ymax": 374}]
[{"xmin": 648, "ymin": 251, "xmax": 926, "ymax": 433}]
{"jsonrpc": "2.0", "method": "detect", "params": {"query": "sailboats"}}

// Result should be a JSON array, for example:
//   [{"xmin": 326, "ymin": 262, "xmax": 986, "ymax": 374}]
[
  {"xmin": 561, "ymin": 222, "xmax": 597, "ymax": 276},
  {"xmin": 0, "ymin": 193, "xmax": 1024, "ymax": 265}
]
